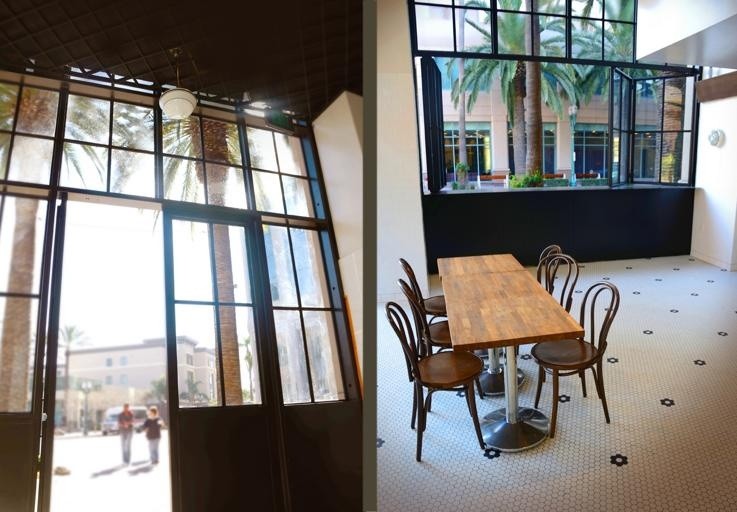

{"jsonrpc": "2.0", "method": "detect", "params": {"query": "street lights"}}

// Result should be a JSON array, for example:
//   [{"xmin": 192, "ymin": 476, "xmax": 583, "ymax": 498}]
[
  {"xmin": 80, "ymin": 381, "xmax": 91, "ymax": 435},
  {"xmin": 196, "ymin": 394, "xmax": 203, "ymax": 403},
  {"xmin": 567, "ymin": 104, "xmax": 577, "ymax": 187}
]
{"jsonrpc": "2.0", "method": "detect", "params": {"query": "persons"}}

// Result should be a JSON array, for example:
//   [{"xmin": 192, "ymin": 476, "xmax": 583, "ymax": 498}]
[
  {"xmin": 136, "ymin": 405, "xmax": 164, "ymax": 464},
  {"xmin": 118, "ymin": 402, "xmax": 134, "ymax": 463}
]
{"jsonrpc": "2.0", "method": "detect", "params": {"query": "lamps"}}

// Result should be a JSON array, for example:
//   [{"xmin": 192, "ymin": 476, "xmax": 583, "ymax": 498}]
[
  {"xmin": 158, "ymin": 46, "xmax": 198, "ymax": 121},
  {"xmin": 707, "ymin": 129, "xmax": 725, "ymax": 147}
]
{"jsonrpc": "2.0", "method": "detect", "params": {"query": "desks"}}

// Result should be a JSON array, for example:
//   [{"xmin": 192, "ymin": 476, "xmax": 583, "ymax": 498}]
[{"xmin": 435, "ymin": 253, "xmax": 584, "ymax": 453}]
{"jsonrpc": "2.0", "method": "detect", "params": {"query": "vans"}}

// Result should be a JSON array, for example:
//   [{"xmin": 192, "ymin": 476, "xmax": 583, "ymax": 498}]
[{"xmin": 101, "ymin": 406, "xmax": 148, "ymax": 436}]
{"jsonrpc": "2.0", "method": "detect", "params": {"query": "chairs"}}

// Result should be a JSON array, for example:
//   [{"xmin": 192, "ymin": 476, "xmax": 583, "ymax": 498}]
[
  {"xmin": 534, "ymin": 244, "xmax": 620, "ymax": 438},
  {"xmin": 384, "ymin": 258, "xmax": 486, "ymax": 462}
]
